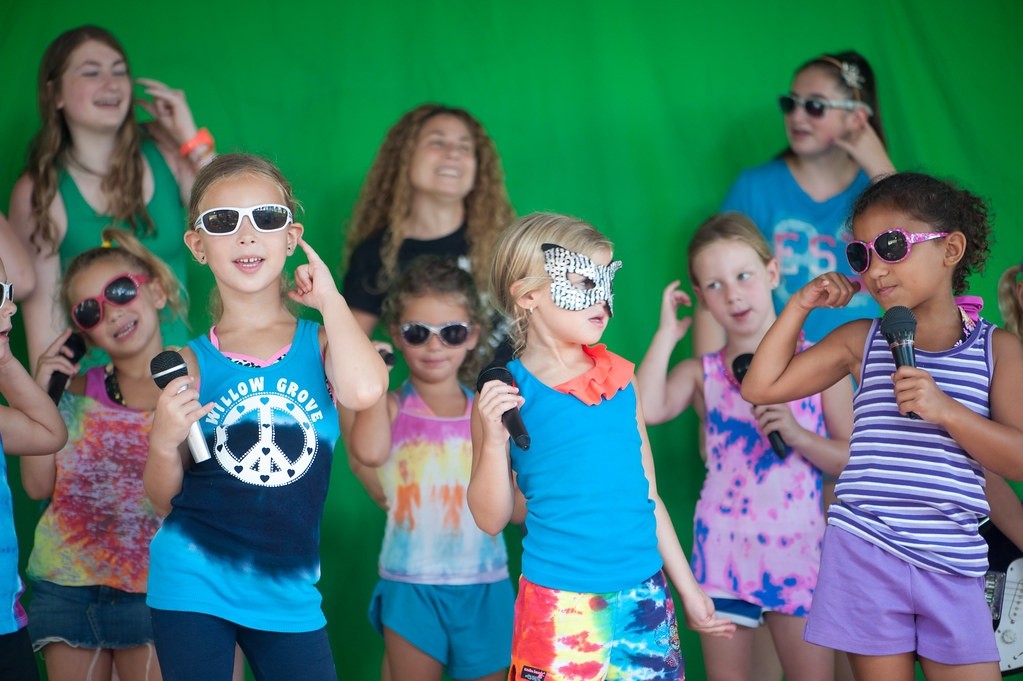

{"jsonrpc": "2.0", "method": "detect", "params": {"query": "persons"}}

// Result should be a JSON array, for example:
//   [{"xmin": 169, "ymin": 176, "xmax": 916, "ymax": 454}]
[
  {"xmin": 339, "ymin": 103, "xmax": 527, "ymax": 681},
  {"xmin": 740, "ymin": 171, "xmax": 1023, "ymax": 680},
  {"xmin": 637, "ymin": 212, "xmax": 854, "ymax": 681},
  {"xmin": 142, "ymin": 155, "xmax": 389, "ymax": 681},
  {"xmin": 984, "ymin": 263, "xmax": 1023, "ymax": 550},
  {"xmin": 22, "ymin": 228, "xmax": 184, "ymax": 681},
  {"xmin": 723, "ymin": 52, "xmax": 897, "ymax": 681},
  {"xmin": 467, "ymin": 215, "xmax": 736, "ymax": 681},
  {"xmin": 1, "ymin": 24, "xmax": 217, "ymax": 681}
]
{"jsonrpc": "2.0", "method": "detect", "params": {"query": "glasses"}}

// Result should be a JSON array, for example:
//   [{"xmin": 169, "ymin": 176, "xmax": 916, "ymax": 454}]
[
  {"xmin": 194, "ymin": 203, "xmax": 293, "ymax": 236},
  {"xmin": 779, "ymin": 96, "xmax": 874, "ymax": 120},
  {"xmin": 398, "ymin": 320, "xmax": 472, "ymax": 349},
  {"xmin": 0, "ymin": 282, "xmax": 14, "ymax": 309},
  {"xmin": 845, "ymin": 227, "xmax": 948, "ymax": 274},
  {"xmin": 71, "ymin": 272, "xmax": 152, "ymax": 332}
]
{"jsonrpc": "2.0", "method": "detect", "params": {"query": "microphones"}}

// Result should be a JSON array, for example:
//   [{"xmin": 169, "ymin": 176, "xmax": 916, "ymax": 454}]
[
  {"xmin": 378, "ymin": 349, "xmax": 396, "ymax": 365},
  {"xmin": 477, "ymin": 361, "xmax": 531, "ymax": 451},
  {"xmin": 881, "ymin": 306, "xmax": 919, "ymax": 419},
  {"xmin": 150, "ymin": 350, "xmax": 212, "ymax": 466},
  {"xmin": 47, "ymin": 333, "xmax": 86, "ymax": 407},
  {"xmin": 731, "ymin": 353, "xmax": 787, "ymax": 459}
]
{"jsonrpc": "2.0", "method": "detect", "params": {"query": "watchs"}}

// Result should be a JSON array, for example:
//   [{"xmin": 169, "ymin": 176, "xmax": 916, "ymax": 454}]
[{"xmin": 180, "ymin": 127, "xmax": 213, "ymax": 155}]
